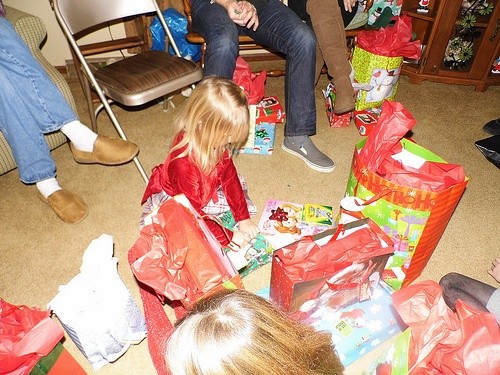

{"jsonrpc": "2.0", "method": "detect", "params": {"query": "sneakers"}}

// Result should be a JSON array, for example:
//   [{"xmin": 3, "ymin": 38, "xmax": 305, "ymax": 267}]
[{"xmin": 284, "ymin": 138, "xmax": 336, "ymax": 173}]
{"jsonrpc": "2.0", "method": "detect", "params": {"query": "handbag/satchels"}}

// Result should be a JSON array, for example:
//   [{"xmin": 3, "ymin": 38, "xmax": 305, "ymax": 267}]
[
  {"xmin": 270, "ymin": 217, "xmax": 395, "ymax": 318},
  {"xmin": 142, "ymin": 195, "xmax": 246, "ymax": 314},
  {"xmin": 18, "ymin": 311, "xmax": 94, "ymax": 375},
  {"xmin": 307, "ymin": 300, "xmax": 411, "ymax": 375},
  {"xmin": 350, "ymin": 43, "xmax": 403, "ymax": 111},
  {"xmin": 339, "ymin": 135, "xmax": 469, "ymax": 290}
]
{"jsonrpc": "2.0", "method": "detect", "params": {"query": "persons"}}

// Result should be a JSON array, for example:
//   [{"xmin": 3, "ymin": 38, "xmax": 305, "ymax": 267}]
[
  {"xmin": 439, "ymin": 259, "xmax": 500, "ymax": 325},
  {"xmin": 164, "ymin": 290, "xmax": 346, "ymax": 375},
  {"xmin": 288, "ymin": 0, "xmax": 359, "ymax": 116},
  {"xmin": 190, "ymin": 0, "xmax": 336, "ymax": 173},
  {"xmin": 0, "ymin": 0, "xmax": 138, "ymax": 224},
  {"xmin": 139, "ymin": 75, "xmax": 260, "ymax": 250}
]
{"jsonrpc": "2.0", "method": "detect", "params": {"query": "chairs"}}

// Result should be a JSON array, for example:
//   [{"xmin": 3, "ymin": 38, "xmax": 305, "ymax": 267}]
[{"xmin": 49, "ymin": 0, "xmax": 373, "ymax": 186}]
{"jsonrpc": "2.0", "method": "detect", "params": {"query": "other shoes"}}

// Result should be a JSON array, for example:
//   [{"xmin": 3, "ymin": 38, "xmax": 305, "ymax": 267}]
[
  {"xmin": 483, "ymin": 118, "xmax": 500, "ymax": 135},
  {"xmin": 70, "ymin": 135, "xmax": 139, "ymax": 165},
  {"xmin": 36, "ymin": 191, "xmax": 89, "ymax": 224}
]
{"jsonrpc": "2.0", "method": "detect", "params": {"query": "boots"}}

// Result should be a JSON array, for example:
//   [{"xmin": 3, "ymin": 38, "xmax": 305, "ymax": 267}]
[
  {"xmin": 306, "ymin": 0, "xmax": 356, "ymax": 114},
  {"xmin": 314, "ymin": 39, "xmax": 324, "ymax": 85}
]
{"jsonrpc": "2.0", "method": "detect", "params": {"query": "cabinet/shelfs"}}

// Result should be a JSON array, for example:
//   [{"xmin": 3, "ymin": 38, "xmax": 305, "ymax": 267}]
[{"xmin": 393, "ymin": 1, "xmax": 500, "ymax": 92}]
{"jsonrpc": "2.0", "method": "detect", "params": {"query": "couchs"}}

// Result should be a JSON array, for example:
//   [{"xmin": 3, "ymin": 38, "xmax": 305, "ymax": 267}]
[{"xmin": 0, "ymin": 5, "xmax": 81, "ymax": 178}]
